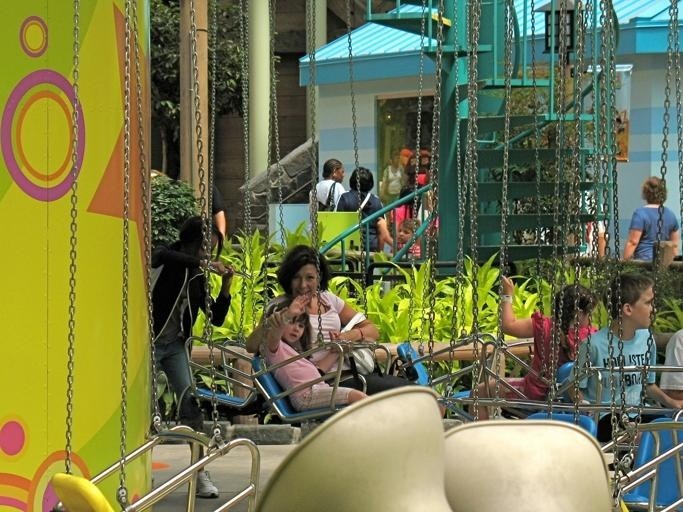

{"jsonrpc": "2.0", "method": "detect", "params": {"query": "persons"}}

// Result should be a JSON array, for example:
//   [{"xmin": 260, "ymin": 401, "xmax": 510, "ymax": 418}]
[
  {"xmin": 573, "ymin": 168, "xmax": 609, "ymax": 279},
  {"xmin": 659, "ymin": 328, "xmax": 683, "ymax": 419},
  {"xmin": 247, "ymin": 244, "xmax": 447, "ymax": 418},
  {"xmin": 259, "ymin": 299, "xmax": 370, "ymax": 412},
  {"xmin": 208, "ymin": 182, "xmax": 227, "ymax": 242},
  {"xmin": 623, "ymin": 176, "xmax": 680, "ymax": 262},
  {"xmin": 567, "ymin": 272, "xmax": 683, "ymax": 472},
  {"xmin": 308, "ymin": 149, "xmax": 439, "ymax": 294},
  {"xmin": 468, "ymin": 274, "xmax": 599, "ymax": 420},
  {"xmin": 149, "ymin": 215, "xmax": 234, "ymax": 438}
]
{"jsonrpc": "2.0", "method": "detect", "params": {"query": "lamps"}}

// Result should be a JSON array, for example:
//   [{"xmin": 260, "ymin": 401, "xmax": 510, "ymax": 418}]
[{"xmin": 536, "ymin": 0, "xmax": 587, "ymax": 76}]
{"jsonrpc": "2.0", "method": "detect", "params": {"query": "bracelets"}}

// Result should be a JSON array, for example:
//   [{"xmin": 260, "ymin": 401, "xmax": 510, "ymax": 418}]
[{"xmin": 357, "ymin": 328, "xmax": 364, "ymax": 343}]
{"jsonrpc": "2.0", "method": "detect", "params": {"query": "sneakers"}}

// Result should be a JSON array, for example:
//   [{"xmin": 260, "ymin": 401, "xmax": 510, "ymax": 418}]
[{"xmin": 197, "ymin": 471, "xmax": 219, "ymax": 497}]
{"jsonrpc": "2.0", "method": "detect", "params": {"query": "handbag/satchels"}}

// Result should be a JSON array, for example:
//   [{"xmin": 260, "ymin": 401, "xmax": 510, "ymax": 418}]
[{"xmin": 345, "ymin": 343, "xmax": 375, "ymax": 376}]
